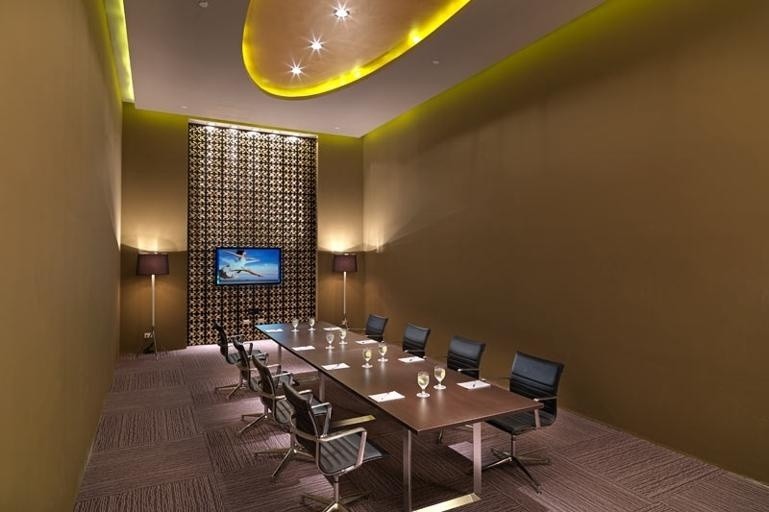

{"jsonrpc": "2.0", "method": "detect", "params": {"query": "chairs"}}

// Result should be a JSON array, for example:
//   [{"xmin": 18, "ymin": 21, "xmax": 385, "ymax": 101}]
[{"xmin": 212, "ymin": 317, "xmax": 391, "ymax": 512}]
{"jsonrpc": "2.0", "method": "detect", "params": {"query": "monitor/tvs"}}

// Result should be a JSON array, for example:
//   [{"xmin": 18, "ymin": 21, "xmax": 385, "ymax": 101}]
[{"xmin": 215, "ymin": 246, "xmax": 282, "ymax": 286}]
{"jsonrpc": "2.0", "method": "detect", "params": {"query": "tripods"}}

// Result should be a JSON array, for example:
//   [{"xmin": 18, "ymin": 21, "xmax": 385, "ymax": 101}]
[
  {"xmin": 336, "ymin": 272, "xmax": 366, "ymax": 333},
  {"xmin": 132, "ymin": 274, "xmax": 170, "ymax": 361}
]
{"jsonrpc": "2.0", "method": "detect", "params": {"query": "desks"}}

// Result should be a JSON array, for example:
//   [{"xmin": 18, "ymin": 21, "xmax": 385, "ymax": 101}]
[
  {"xmin": 254, "ymin": 320, "xmax": 545, "ymax": 512},
  {"xmin": 357, "ymin": 312, "xmax": 565, "ymax": 495}
]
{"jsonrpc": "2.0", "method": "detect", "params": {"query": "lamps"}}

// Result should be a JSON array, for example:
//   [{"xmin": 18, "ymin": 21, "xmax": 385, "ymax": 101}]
[
  {"xmin": 332, "ymin": 251, "xmax": 358, "ymax": 329},
  {"xmin": 133, "ymin": 252, "xmax": 170, "ymax": 360}
]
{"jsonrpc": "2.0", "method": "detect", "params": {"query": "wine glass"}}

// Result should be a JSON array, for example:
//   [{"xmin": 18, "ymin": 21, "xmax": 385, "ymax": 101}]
[
  {"xmin": 326, "ymin": 330, "xmax": 349, "ymax": 350},
  {"xmin": 415, "ymin": 365, "xmax": 447, "ymax": 397},
  {"xmin": 291, "ymin": 318, "xmax": 318, "ymax": 332},
  {"xmin": 361, "ymin": 342, "xmax": 389, "ymax": 369}
]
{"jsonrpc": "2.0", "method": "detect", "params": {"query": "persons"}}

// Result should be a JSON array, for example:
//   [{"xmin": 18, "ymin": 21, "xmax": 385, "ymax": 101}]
[{"xmin": 223, "ymin": 248, "xmax": 264, "ymax": 278}]
